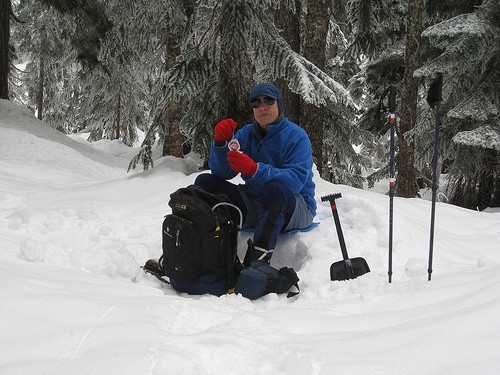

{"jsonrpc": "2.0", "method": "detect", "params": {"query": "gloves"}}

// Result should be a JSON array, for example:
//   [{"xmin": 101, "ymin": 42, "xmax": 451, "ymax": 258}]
[
  {"xmin": 214, "ymin": 119, "xmax": 237, "ymax": 142},
  {"xmin": 227, "ymin": 150, "xmax": 258, "ymax": 177}
]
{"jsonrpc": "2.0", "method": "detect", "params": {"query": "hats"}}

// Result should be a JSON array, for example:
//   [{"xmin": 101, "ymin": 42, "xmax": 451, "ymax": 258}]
[{"xmin": 249, "ymin": 83, "xmax": 283, "ymax": 113}]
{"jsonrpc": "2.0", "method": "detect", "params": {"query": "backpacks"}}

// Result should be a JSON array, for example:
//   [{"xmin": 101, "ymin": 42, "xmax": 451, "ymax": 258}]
[{"xmin": 162, "ymin": 183, "xmax": 245, "ymax": 296}]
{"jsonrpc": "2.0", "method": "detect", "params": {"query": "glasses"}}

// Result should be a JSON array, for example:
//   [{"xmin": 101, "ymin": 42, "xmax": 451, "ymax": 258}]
[{"xmin": 250, "ymin": 95, "xmax": 276, "ymax": 109}]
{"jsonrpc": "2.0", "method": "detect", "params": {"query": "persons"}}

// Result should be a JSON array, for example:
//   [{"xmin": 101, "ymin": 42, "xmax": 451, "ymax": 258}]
[{"xmin": 194, "ymin": 83, "xmax": 317, "ymax": 268}]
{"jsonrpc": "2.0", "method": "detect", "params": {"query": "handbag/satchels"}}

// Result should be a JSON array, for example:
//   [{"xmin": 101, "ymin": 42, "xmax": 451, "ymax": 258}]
[{"xmin": 237, "ymin": 262, "xmax": 301, "ymax": 299}]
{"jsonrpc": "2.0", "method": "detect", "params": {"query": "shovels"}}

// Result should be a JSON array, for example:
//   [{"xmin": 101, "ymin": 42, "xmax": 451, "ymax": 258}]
[{"xmin": 320, "ymin": 193, "xmax": 371, "ymax": 281}]
